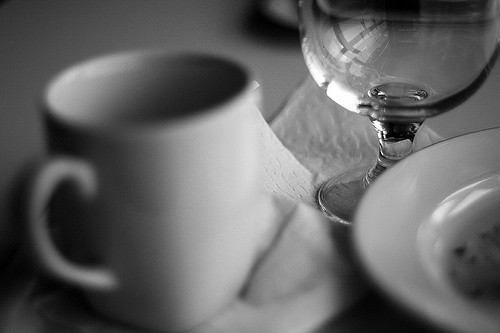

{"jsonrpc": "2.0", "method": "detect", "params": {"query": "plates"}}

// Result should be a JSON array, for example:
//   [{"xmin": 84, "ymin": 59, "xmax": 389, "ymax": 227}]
[{"xmin": 350, "ymin": 125, "xmax": 499, "ymax": 333}]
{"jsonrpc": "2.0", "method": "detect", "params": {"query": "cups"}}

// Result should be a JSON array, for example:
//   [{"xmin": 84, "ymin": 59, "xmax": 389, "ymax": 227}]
[{"xmin": 21, "ymin": 47, "xmax": 268, "ymax": 332}]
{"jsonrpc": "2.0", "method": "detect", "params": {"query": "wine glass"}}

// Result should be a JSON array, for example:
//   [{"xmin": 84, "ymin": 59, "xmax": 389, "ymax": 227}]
[{"xmin": 289, "ymin": 0, "xmax": 499, "ymax": 228}]
